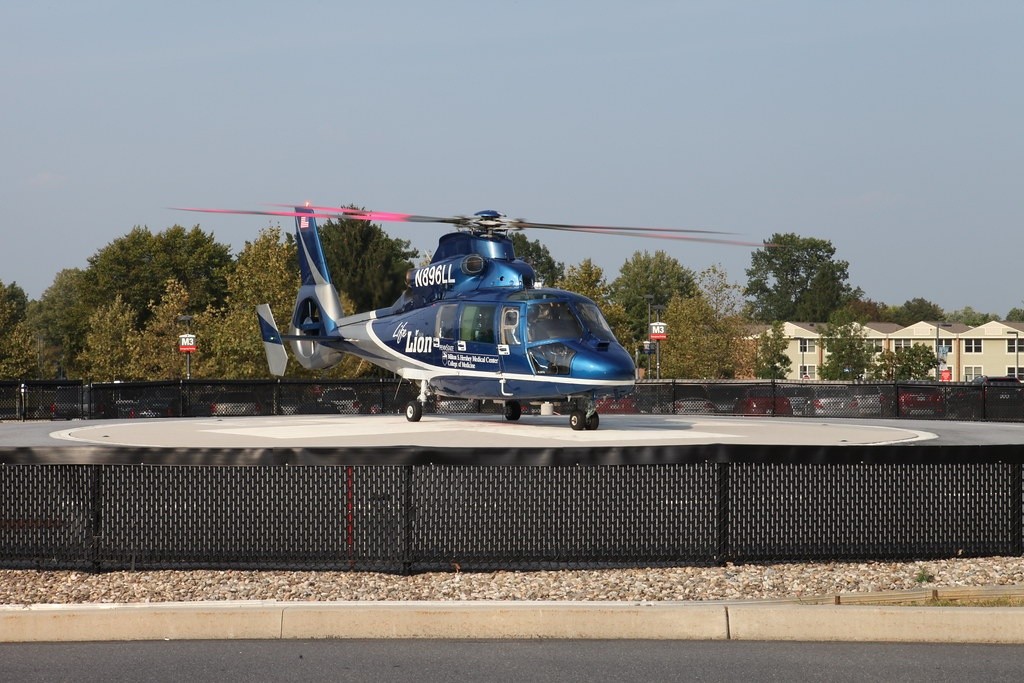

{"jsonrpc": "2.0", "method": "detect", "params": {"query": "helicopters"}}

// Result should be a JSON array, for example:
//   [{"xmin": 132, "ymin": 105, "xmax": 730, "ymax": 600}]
[{"xmin": 171, "ymin": 200, "xmax": 781, "ymax": 430}]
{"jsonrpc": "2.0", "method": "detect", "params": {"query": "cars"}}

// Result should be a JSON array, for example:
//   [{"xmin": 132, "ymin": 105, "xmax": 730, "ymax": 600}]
[{"xmin": 0, "ymin": 374, "xmax": 1024, "ymax": 419}]
